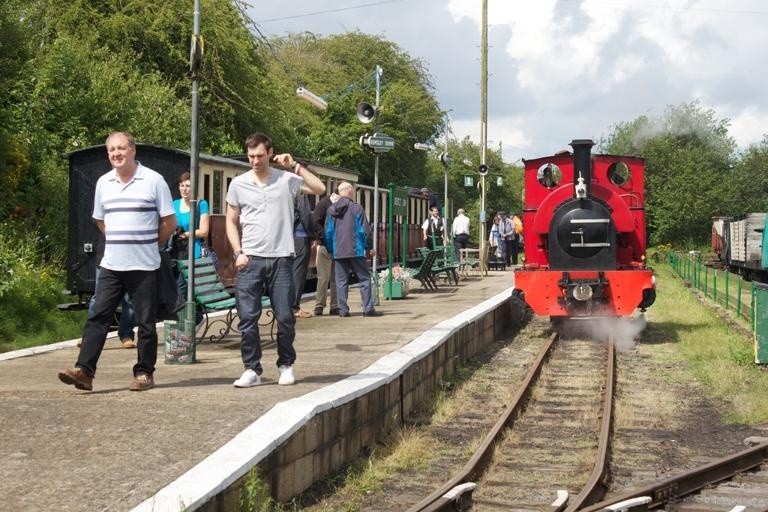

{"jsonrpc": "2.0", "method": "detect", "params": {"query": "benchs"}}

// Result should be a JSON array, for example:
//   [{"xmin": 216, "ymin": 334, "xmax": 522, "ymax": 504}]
[
  {"xmin": 175, "ymin": 258, "xmax": 278, "ymax": 350},
  {"xmin": 405, "ymin": 242, "xmax": 505, "ymax": 293}
]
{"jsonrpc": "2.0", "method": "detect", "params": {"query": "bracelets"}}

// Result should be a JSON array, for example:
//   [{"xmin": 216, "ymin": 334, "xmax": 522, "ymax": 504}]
[
  {"xmin": 291, "ymin": 160, "xmax": 296, "ymax": 171},
  {"xmin": 233, "ymin": 251, "xmax": 243, "ymax": 260},
  {"xmin": 295, "ymin": 163, "xmax": 300, "ymax": 175}
]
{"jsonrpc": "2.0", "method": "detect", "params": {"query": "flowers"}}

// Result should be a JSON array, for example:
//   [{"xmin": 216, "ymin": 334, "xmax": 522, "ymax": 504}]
[{"xmin": 377, "ymin": 265, "xmax": 415, "ymax": 284}]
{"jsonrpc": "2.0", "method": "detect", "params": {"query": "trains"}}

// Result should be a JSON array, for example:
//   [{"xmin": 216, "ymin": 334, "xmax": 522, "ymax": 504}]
[
  {"xmin": 707, "ymin": 205, "xmax": 767, "ymax": 285},
  {"xmin": 513, "ymin": 139, "xmax": 657, "ymax": 325},
  {"xmin": 53, "ymin": 142, "xmax": 450, "ymax": 335}
]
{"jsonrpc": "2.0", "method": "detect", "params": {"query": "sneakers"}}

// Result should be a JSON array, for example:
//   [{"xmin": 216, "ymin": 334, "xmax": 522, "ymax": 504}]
[
  {"xmin": 294, "ymin": 307, "xmax": 384, "ymax": 318},
  {"xmin": 121, "ymin": 338, "xmax": 134, "ymax": 349},
  {"xmin": 278, "ymin": 362, "xmax": 295, "ymax": 385},
  {"xmin": 58, "ymin": 369, "xmax": 93, "ymax": 391},
  {"xmin": 234, "ymin": 366, "xmax": 262, "ymax": 388},
  {"xmin": 129, "ymin": 371, "xmax": 155, "ymax": 391}
]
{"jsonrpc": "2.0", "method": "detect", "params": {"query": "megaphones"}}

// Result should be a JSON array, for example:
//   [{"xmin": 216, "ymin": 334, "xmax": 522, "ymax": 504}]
[
  {"xmin": 477, "ymin": 164, "xmax": 490, "ymax": 175},
  {"xmin": 356, "ymin": 101, "xmax": 380, "ymax": 124}
]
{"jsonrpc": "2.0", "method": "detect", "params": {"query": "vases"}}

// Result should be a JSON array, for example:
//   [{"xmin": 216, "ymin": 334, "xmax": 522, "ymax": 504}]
[{"xmin": 382, "ymin": 280, "xmax": 410, "ymax": 299}]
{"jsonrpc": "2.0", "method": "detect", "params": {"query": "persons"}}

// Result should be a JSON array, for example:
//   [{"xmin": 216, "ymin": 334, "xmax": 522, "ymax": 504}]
[
  {"xmin": 323, "ymin": 181, "xmax": 384, "ymax": 318},
  {"xmin": 224, "ymin": 132, "xmax": 326, "ymax": 389},
  {"xmin": 421, "ymin": 206, "xmax": 449, "ymax": 267},
  {"xmin": 57, "ymin": 133, "xmax": 177, "ymax": 392},
  {"xmin": 452, "ymin": 208, "xmax": 470, "ymax": 260},
  {"xmin": 291, "ymin": 167, "xmax": 318, "ymax": 318},
  {"xmin": 488, "ymin": 210, "xmax": 523, "ymax": 267},
  {"xmin": 76, "ymin": 208, "xmax": 136, "ymax": 349},
  {"xmin": 312, "ymin": 188, "xmax": 341, "ymax": 315},
  {"xmin": 170, "ymin": 171, "xmax": 210, "ymax": 334}
]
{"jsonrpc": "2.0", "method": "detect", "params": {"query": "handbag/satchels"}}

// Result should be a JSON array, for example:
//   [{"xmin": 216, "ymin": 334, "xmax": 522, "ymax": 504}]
[{"xmin": 200, "ymin": 244, "xmax": 220, "ymax": 270}]
